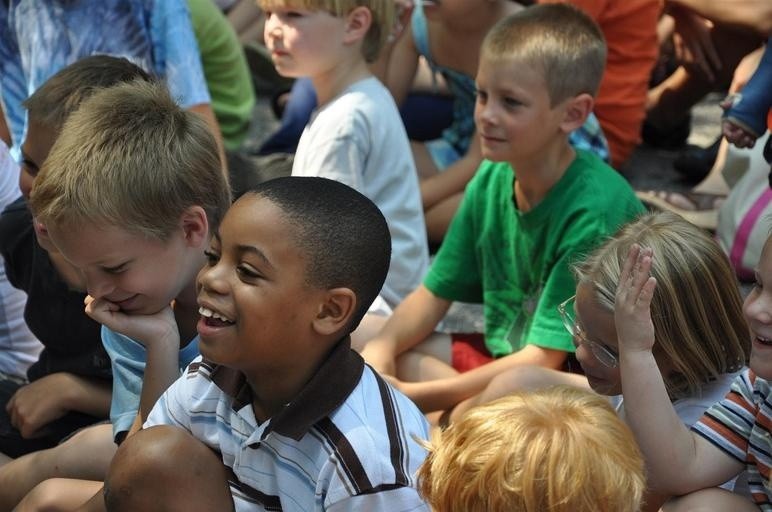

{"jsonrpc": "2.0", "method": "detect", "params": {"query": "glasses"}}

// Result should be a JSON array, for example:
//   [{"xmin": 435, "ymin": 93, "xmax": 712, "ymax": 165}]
[{"xmin": 558, "ymin": 294, "xmax": 619, "ymax": 368}]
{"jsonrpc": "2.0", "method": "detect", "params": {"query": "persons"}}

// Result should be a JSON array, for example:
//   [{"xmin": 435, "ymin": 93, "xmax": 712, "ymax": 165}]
[
  {"xmin": 1, "ymin": 1, "xmax": 771, "ymax": 421},
  {"xmin": 1, "ymin": 79, "xmax": 234, "ymax": 509},
  {"xmin": 103, "ymin": 176, "xmax": 434, "ymax": 512},
  {"xmin": 414, "ymin": 385, "xmax": 644, "ymax": 511},
  {"xmin": 613, "ymin": 229, "xmax": 771, "ymax": 511}
]
